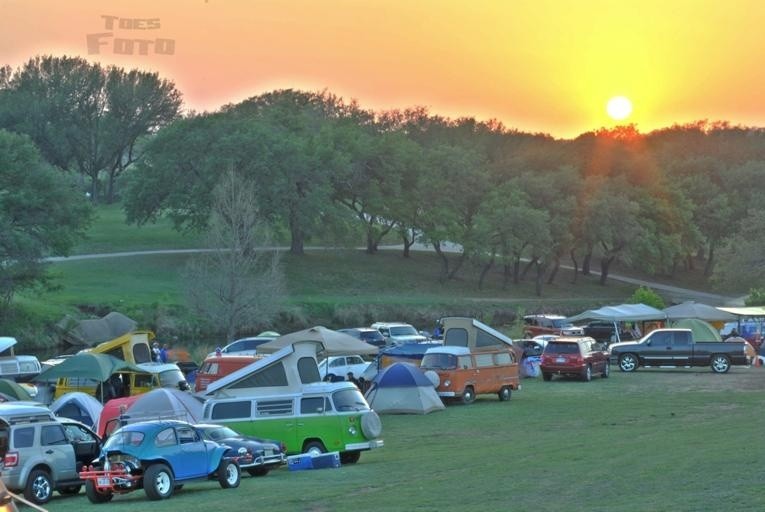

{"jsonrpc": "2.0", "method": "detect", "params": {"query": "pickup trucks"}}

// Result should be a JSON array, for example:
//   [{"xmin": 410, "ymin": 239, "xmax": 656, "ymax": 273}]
[{"xmin": 606, "ymin": 326, "xmax": 755, "ymax": 377}]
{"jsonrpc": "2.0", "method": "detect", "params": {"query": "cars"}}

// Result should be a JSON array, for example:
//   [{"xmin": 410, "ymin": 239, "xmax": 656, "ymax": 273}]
[
  {"xmin": 0, "ymin": 316, "xmax": 285, "ymax": 509},
  {"xmin": 321, "ymin": 299, "xmax": 643, "ymax": 411}
]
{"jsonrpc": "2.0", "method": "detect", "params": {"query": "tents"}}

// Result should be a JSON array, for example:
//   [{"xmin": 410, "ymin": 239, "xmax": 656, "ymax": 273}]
[
  {"xmin": 365, "ymin": 361, "xmax": 446, "ymax": 414},
  {"xmin": 671, "ymin": 319, "xmax": 722, "ymax": 343},
  {"xmin": 0, "ymin": 314, "xmax": 214, "ymax": 450}
]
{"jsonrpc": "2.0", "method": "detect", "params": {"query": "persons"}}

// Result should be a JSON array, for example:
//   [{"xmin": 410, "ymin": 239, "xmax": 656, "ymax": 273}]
[
  {"xmin": 434, "ymin": 319, "xmax": 444, "ymax": 334},
  {"xmin": 347, "ymin": 371, "xmax": 362, "ymax": 394},
  {"xmin": 727, "ymin": 328, "xmax": 738, "ymax": 338}
]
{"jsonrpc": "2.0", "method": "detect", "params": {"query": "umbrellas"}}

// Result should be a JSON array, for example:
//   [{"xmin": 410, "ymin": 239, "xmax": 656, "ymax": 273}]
[
  {"xmin": 255, "ymin": 324, "xmax": 381, "ymax": 384},
  {"xmin": 664, "ymin": 300, "xmax": 739, "ymax": 321}
]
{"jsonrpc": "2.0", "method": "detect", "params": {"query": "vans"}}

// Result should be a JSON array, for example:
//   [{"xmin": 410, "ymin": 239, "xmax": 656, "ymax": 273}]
[
  {"xmin": 202, "ymin": 379, "xmax": 383, "ymax": 464},
  {"xmin": 192, "ymin": 354, "xmax": 271, "ymax": 397},
  {"xmin": 55, "ymin": 363, "xmax": 190, "ymax": 403},
  {"xmin": 418, "ymin": 342, "xmax": 529, "ymax": 400}
]
